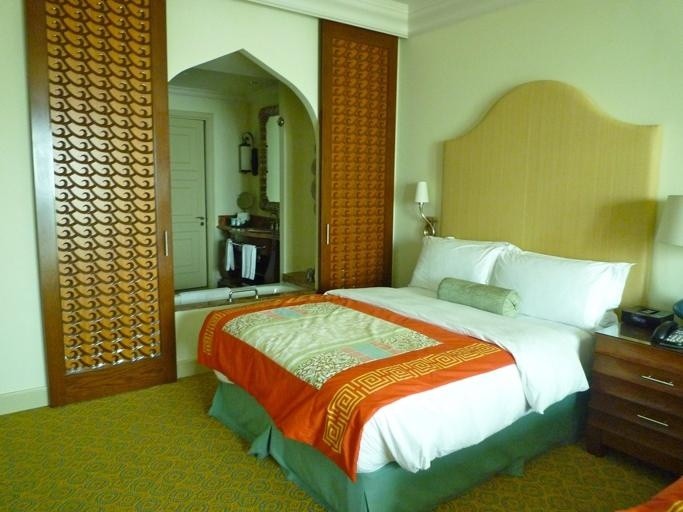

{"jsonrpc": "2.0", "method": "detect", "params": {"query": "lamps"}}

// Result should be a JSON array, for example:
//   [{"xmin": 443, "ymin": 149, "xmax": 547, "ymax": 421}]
[
  {"xmin": 414, "ymin": 181, "xmax": 437, "ymax": 236},
  {"xmin": 655, "ymin": 194, "xmax": 682, "ymax": 245}
]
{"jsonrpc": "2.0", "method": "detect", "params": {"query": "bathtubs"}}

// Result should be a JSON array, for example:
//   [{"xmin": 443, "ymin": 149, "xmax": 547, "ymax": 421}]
[{"xmin": 174, "ymin": 281, "xmax": 304, "ymax": 306}]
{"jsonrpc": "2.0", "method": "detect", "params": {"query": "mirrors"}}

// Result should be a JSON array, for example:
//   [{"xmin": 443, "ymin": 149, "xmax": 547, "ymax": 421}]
[{"xmin": 258, "ymin": 106, "xmax": 281, "ymax": 211}]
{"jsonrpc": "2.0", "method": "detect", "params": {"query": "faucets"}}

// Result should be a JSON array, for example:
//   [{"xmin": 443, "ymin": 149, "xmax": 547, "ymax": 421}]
[
  {"xmin": 271, "ymin": 210, "xmax": 280, "ymax": 231},
  {"xmin": 305, "ymin": 268, "xmax": 315, "ymax": 282}
]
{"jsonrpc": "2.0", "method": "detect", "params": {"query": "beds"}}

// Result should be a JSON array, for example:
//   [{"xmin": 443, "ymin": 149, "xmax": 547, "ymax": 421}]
[{"xmin": 210, "ymin": 286, "xmax": 620, "ymax": 512}]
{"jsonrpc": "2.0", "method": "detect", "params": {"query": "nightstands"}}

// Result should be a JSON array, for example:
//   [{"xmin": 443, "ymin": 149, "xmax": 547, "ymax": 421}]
[{"xmin": 586, "ymin": 319, "xmax": 682, "ymax": 480}]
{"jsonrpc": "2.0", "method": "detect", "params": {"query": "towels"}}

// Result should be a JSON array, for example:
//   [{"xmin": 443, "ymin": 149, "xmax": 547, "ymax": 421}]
[{"xmin": 224, "ymin": 238, "xmax": 256, "ymax": 280}]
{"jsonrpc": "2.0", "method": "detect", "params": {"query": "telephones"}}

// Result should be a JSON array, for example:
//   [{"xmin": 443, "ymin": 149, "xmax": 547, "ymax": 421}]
[{"xmin": 651, "ymin": 320, "xmax": 683, "ymax": 349}]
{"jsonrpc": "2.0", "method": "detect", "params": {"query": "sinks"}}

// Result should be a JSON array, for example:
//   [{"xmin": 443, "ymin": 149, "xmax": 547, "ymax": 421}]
[{"xmin": 247, "ymin": 227, "xmax": 274, "ymax": 233}]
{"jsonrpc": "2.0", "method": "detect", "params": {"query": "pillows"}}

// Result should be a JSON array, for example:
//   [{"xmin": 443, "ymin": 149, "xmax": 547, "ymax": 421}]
[{"xmin": 410, "ymin": 236, "xmax": 635, "ymax": 330}]
